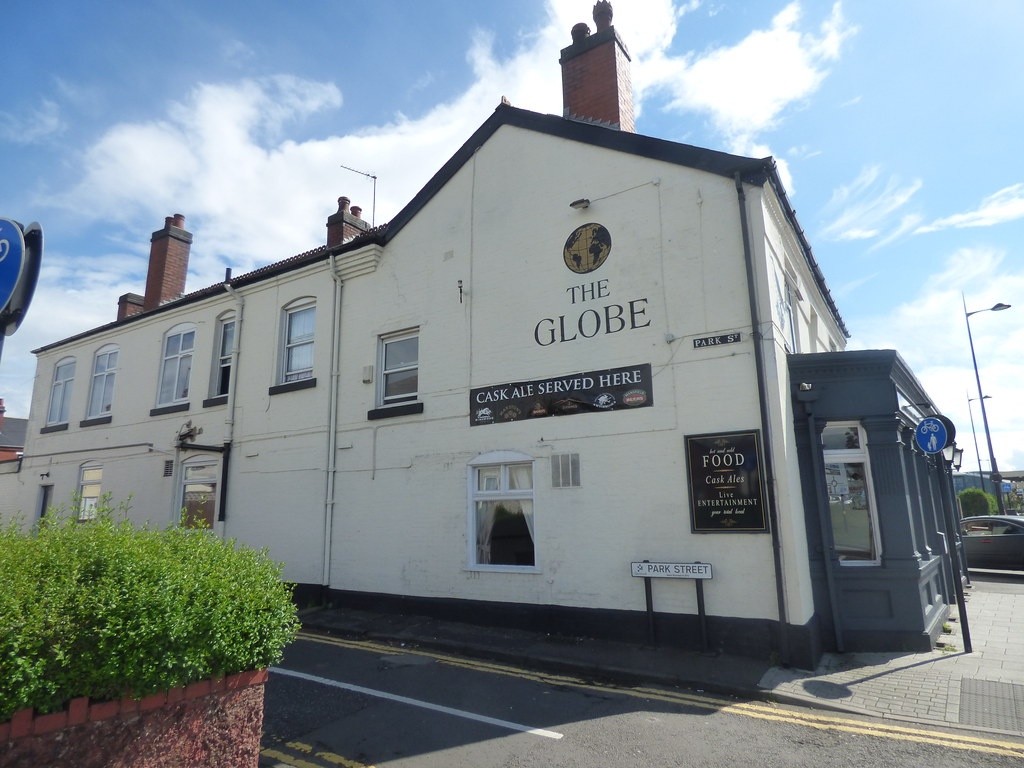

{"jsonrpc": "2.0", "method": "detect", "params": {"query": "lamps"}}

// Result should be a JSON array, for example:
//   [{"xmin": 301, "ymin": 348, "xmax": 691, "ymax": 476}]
[
  {"xmin": 942, "ymin": 448, "xmax": 964, "ymax": 473},
  {"xmin": 927, "ymin": 440, "xmax": 958, "ymax": 475},
  {"xmin": 570, "ymin": 199, "xmax": 590, "ymax": 210},
  {"xmin": 912, "ymin": 402, "xmax": 930, "ymax": 408}
]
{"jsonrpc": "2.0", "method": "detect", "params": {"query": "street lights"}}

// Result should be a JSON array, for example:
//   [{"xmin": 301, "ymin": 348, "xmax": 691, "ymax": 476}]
[
  {"xmin": 968, "ymin": 396, "xmax": 993, "ymax": 493},
  {"xmin": 966, "ymin": 303, "xmax": 1012, "ymax": 515}
]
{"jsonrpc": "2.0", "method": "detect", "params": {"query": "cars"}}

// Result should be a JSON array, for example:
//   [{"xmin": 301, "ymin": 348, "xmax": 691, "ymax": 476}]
[{"xmin": 960, "ymin": 515, "xmax": 1024, "ymax": 575}]
